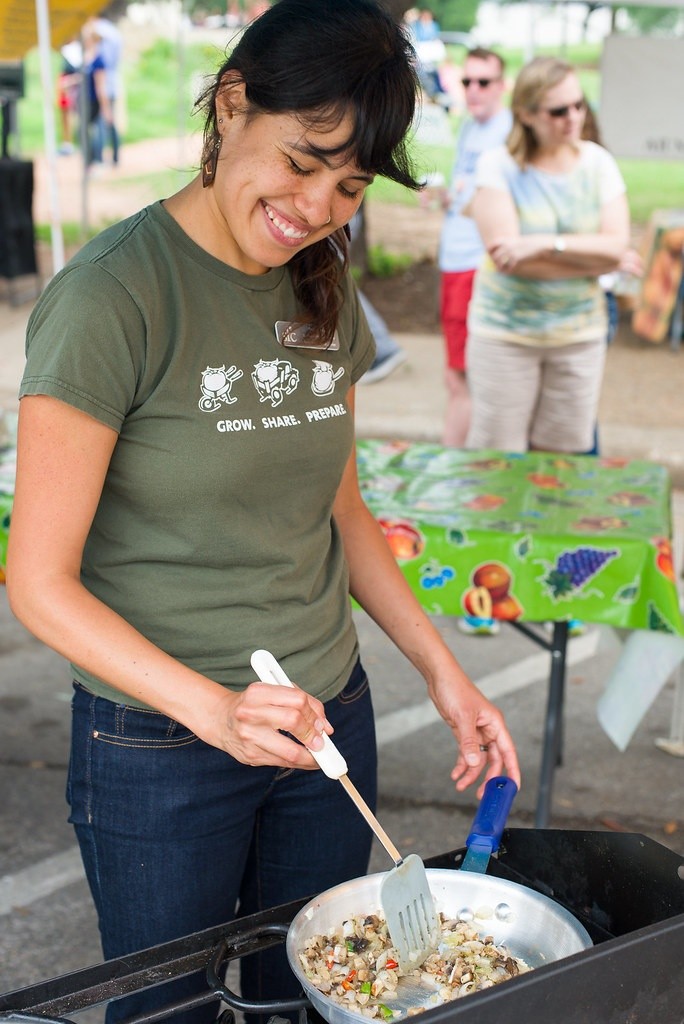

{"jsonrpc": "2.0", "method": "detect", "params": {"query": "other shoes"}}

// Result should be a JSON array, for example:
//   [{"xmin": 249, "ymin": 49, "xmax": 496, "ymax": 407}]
[
  {"xmin": 459, "ymin": 617, "xmax": 501, "ymax": 636},
  {"xmin": 546, "ymin": 620, "xmax": 586, "ymax": 636}
]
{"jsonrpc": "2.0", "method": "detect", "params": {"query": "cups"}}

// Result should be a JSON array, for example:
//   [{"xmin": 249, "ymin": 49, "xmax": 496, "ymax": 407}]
[{"xmin": 421, "ymin": 174, "xmax": 444, "ymax": 212}]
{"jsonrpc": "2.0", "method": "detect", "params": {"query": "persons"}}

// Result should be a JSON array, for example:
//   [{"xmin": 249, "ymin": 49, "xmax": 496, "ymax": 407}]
[
  {"xmin": 342, "ymin": 204, "xmax": 407, "ymax": 383},
  {"xmin": 466, "ymin": 56, "xmax": 631, "ymax": 454},
  {"xmin": 60, "ymin": 22, "xmax": 122, "ymax": 180},
  {"xmin": 430, "ymin": 47, "xmax": 516, "ymax": 447},
  {"xmin": 8, "ymin": 1, "xmax": 519, "ymax": 1024},
  {"xmin": 402, "ymin": 8, "xmax": 451, "ymax": 112}
]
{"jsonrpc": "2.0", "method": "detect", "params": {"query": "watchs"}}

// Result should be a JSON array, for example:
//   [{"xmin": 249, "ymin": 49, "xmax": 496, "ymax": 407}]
[{"xmin": 550, "ymin": 237, "xmax": 566, "ymax": 262}]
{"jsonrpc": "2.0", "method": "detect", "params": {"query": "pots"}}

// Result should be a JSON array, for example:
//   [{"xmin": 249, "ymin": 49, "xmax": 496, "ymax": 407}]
[{"xmin": 286, "ymin": 776, "xmax": 593, "ymax": 1023}]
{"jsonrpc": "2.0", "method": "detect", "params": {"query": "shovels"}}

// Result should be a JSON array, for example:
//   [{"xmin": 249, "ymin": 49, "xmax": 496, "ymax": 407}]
[{"xmin": 251, "ymin": 648, "xmax": 443, "ymax": 976}]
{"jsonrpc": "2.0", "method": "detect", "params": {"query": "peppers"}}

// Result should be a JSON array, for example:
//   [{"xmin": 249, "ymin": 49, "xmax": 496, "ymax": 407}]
[{"xmin": 326, "ymin": 943, "xmax": 399, "ymax": 1017}]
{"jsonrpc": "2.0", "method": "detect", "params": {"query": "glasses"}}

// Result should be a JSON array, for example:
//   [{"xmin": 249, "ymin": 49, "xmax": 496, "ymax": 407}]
[
  {"xmin": 548, "ymin": 102, "xmax": 584, "ymax": 118},
  {"xmin": 459, "ymin": 77, "xmax": 491, "ymax": 87}
]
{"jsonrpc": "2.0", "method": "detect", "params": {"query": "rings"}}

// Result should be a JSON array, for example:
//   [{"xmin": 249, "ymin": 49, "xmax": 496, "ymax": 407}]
[{"xmin": 480, "ymin": 745, "xmax": 489, "ymax": 751}]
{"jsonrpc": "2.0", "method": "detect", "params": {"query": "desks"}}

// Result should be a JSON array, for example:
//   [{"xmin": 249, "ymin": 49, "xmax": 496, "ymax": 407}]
[{"xmin": 0, "ymin": 409, "xmax": 684, "ymax": 832}]
